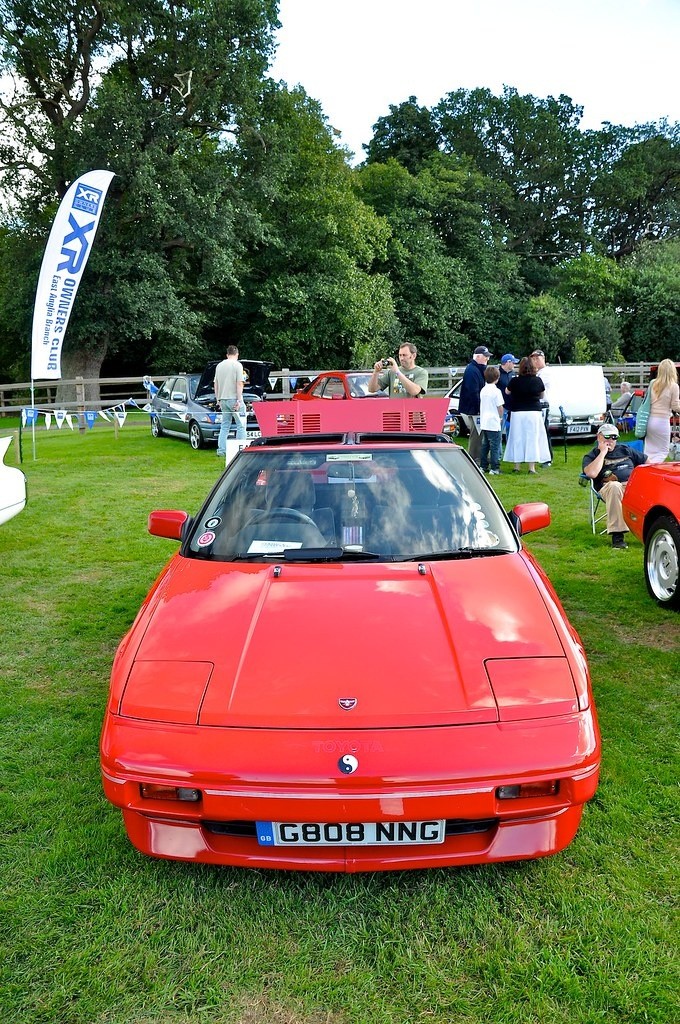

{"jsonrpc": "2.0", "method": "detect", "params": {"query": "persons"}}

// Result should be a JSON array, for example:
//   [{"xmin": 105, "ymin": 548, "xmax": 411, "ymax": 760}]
[
  {"xmin": 581, "ymin": 424, "xmax": 652, "ymax": 548},
  {"xmin": 368, "ymin": 343, "xmax": 429, "ymax": 431},
  {"xmin": 458, "ymin": 346, "xmax": 554, "ymax": 475},
  {"xmin": 214, "ymin": 345, "xmax": 247, "ymax": 457},
  {"xmin": 604, "ymin": 377, "xmax": 632, "ymax": 417},
  {"xmin": 642, "ymin": 358, "xmax": 680, "ymax": 465}
]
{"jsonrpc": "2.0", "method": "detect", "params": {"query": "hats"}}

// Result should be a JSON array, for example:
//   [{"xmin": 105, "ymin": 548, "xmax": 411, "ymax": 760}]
[
  {"xmin": 501, "ymin": 354, "xmax": 520, "ymax": 363},
  {"xmin": 530, "ymin": 350, "xmax": 544, "ymax": 356},
  {"xmin": 597, "ymin": 424, "xmax": 620, "ymax": 438},
  {"xmin": 475, "ymin": 346, "xmax": 493, "ymax": 356}
]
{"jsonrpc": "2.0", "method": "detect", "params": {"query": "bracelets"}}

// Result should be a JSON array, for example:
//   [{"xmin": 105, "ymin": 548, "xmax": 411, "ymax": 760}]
[{"xmin": 395, "ymin": 371, "xmax": 401, "ymax": 377}]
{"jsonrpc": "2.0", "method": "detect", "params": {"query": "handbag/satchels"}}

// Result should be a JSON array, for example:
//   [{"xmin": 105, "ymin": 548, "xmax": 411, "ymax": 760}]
[{"xmin": 635, "ymin": 379, "xmax": 656, "ymax": 438}]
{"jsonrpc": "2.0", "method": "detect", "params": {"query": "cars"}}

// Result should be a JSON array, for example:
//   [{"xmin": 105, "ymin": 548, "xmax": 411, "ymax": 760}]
[
  {"xmin": 99, "ymin": 398, "xmax": 602, "ymax": 873},
  {"xmin": 150, "ymin": 360, "xmax": 277, "ymax": 450},
  {"xmin": 444, "ymin": 365, "xmax": 609, "ymax": 444},
  {"xmin": 291, "ymin": 372, "xmax": 460, "ymax": 440},
  {"xmin": 621, "ymin": 462, "xmax": 680, "ymax": 612}
]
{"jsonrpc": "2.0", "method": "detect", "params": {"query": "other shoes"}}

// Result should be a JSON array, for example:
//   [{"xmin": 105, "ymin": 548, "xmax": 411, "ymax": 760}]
[
  {"xmin": 489, "ymin": 470, "xmax": 500, "ymax": 474},
  {"xmin": 612, "ymin": 538, "xmax": 627, "ymax": 548},
  {"xmin": 479, "ymin": 467, "xmax": 489, "ymax": 473},
  {"xmin": 528, "ymin": 470, "xmax": 538, "ymax": 474},
  {"xmin": 540, "ymin": 463, "xmax": 551, "ymax": 468},
  {"xmin": 511, "ymin": 468, "xmax": 520, "ymax": 473}
]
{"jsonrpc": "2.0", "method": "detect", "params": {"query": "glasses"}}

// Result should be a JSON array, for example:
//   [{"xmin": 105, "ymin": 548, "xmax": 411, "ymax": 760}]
[{"xmin": 600, "ymin": 433, "xmax": 618, "ymax": 441}]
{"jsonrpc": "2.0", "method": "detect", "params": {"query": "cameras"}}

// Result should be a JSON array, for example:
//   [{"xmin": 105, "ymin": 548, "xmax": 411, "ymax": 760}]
[{"xmin": 382, "ymin": 360, "xmax": 389, "ymax": 366}]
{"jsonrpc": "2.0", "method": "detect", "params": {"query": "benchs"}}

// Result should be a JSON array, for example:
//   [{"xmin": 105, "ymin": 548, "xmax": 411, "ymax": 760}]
[{"xmin": 252, "ymin": 483, "xmax": 388, "ymax": 537}]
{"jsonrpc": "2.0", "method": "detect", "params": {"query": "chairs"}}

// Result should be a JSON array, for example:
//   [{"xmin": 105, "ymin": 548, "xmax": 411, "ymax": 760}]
[
  {"xmin": 244, "ymin": 470, "xmax": 335, "ymax": 545},
  {"xmin": 612, "ymin": 389, "xmax": 645, "ymax": 430},
  {"xmin": 372, "ymin": 469, "xmax": 461, "ymax": 551},
  {"xmin": 580, "ymin": 440, "xmax": 643, "ymax": 535}
]
